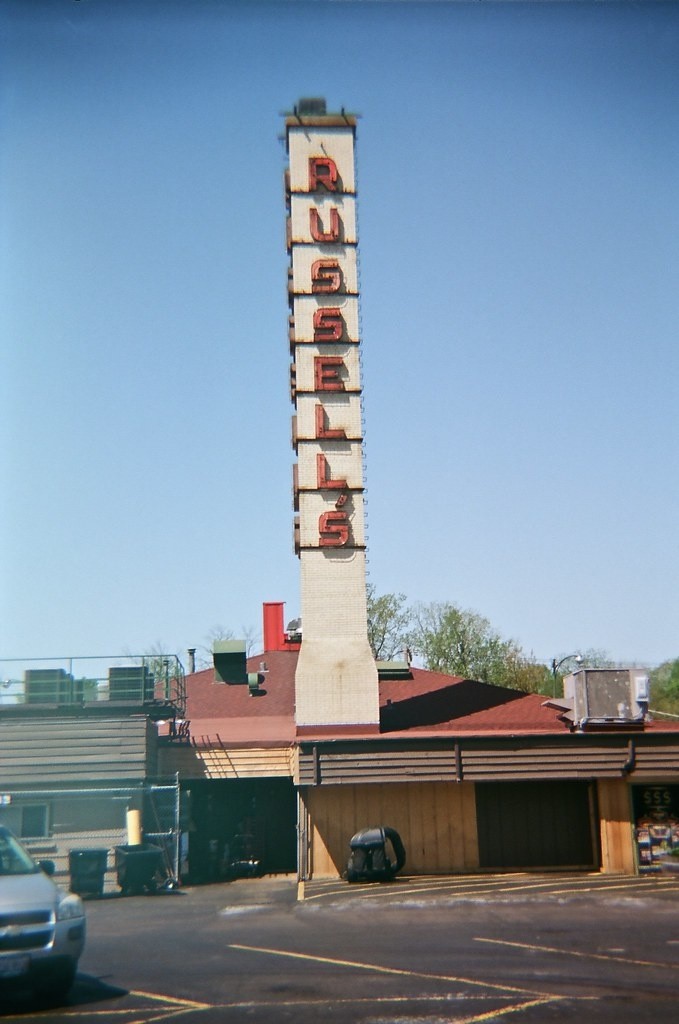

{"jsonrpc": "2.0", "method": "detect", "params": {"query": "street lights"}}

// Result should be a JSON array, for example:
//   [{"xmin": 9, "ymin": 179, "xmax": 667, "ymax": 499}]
[{"xmin": 552, "ymin": 654, "xmax": 584, "ymax": 698}]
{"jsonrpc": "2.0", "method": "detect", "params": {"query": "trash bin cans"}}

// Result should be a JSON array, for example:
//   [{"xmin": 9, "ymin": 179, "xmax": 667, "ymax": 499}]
[
  {"xmin": 113, "ymin": 843, "xmax": 166, "ymax": 892},
  {"xmin": 67, "ymin": 847, "xmax": 112, "ymax": 896}
]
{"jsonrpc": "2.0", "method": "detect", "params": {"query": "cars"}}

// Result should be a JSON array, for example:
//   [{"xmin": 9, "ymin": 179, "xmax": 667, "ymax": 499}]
[{"xmin": 0, "ymin": 823, "xmax": 87, "ymax": 1006}]
